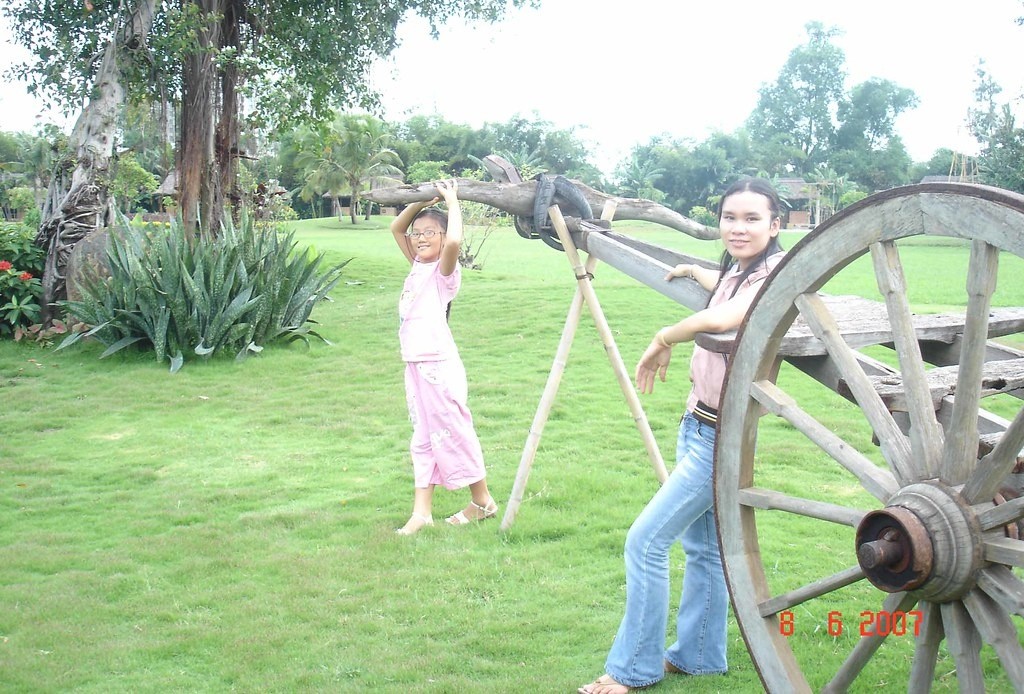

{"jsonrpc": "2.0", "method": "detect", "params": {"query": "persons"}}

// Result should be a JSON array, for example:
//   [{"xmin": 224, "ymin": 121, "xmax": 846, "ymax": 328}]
[
  {"xmin": 389, "ymin": 177, "xmax": 499, "ymax": 538},
  {"xmin": 577, "ymin": 179, "xmax": 789, "ymax": 694}
]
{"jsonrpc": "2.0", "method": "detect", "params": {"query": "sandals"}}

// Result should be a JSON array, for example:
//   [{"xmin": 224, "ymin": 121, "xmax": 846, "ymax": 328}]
[
  {"xmin": 445, "ymin": 494, "xmax": 497, "ymax": 527},
  {"xmin": 396, "ymin": 511, "xmax": 434, "ymax": 536}
]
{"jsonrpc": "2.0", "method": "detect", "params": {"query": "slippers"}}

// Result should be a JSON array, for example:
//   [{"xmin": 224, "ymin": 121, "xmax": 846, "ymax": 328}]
[
  {"xmin": 578, "ymin": 678, "xmax": 647, "ymax": 694},
  {"xmin": 663, "ymin": 657, "xmax": 689, "ymax": 674}
]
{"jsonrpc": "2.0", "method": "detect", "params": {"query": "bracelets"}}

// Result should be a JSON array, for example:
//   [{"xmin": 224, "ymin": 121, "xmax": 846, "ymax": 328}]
[
  {"xmin": 689, "ymin": 264, "xmax": 697, "ymax": 280},
  {"xmin": 658, "ymin": 326, "xmax": 677, "ymax": 348}
]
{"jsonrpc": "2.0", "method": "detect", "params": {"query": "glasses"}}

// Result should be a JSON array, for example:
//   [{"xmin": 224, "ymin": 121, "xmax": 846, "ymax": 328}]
[{"xmin": 409, "ymin": 230, "xmax": 445, "ymax": 239}]
